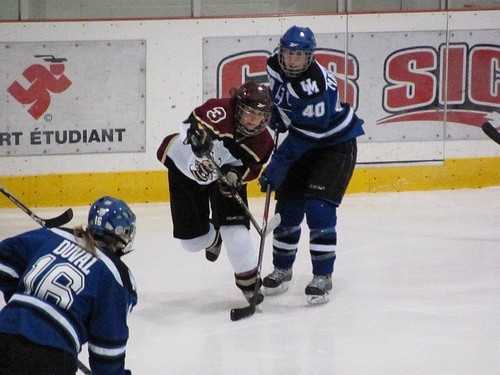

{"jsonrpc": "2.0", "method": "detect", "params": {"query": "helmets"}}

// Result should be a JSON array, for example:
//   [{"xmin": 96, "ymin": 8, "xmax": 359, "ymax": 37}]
[
  {"xmin": 88, "ymin": 196, "xmax": 137, "ymax": 248},
  {"xmin": 279, "ymin": 26, "xmax": 316, "ymax": 77},
  {"xmin": 234, "ymin": 81, "xmax": 272, "ymax": 137}
]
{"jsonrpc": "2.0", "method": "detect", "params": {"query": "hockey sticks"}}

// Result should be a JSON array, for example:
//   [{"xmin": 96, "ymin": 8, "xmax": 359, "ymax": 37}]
[
  {"xmin": 230, "ymin": 118, "xmax": 282, "ymax": 322},
  {"xmin": 209, "ymin": 151, "xmax": 261, "ymax": 235},
  {"xmin": 78, "ymin": 359, "xmax": 92, "ymax": 375},
  {"xmin": 1, "ymin": 188, "xmax": 73, "ymax": 227},
  {"xmin": 482, "ymin": 120, "xmax": 500, "ymax": 144}
]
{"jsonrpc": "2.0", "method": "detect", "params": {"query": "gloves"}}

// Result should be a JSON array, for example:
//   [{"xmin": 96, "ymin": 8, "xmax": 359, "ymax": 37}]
[{"xmin": 219, "ymin": 172, "xmax": 241, "ymax": 197}]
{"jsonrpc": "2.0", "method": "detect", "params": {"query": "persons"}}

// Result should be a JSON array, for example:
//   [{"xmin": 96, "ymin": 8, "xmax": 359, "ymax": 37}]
[
  {"xmin": 0, "ymin": 195, "xmax": 140, "ymax": 375},
  {"xmin": 258, "ymin": 24, "xmax": 366, "ymax": 297},
  {"xmin": 167, "ymin": 81, "xmax": 276, "ymax": 306}
]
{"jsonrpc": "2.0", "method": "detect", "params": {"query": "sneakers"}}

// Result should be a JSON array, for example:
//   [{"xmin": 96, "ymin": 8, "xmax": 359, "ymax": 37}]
[
  {"xmin": 205, "ymin": 230, "xmax": 222, "ymax": 262},
  {"xmin": 305, "ymin": 274, "xmax": 333, "ymax": 304},
  {"xmin": 262, "ymin": 266, "xmax": 292, "ymax": 295},
  {"xmin": 242, "ymin": 289, "xmax": 264, "ymax": 313}
]
